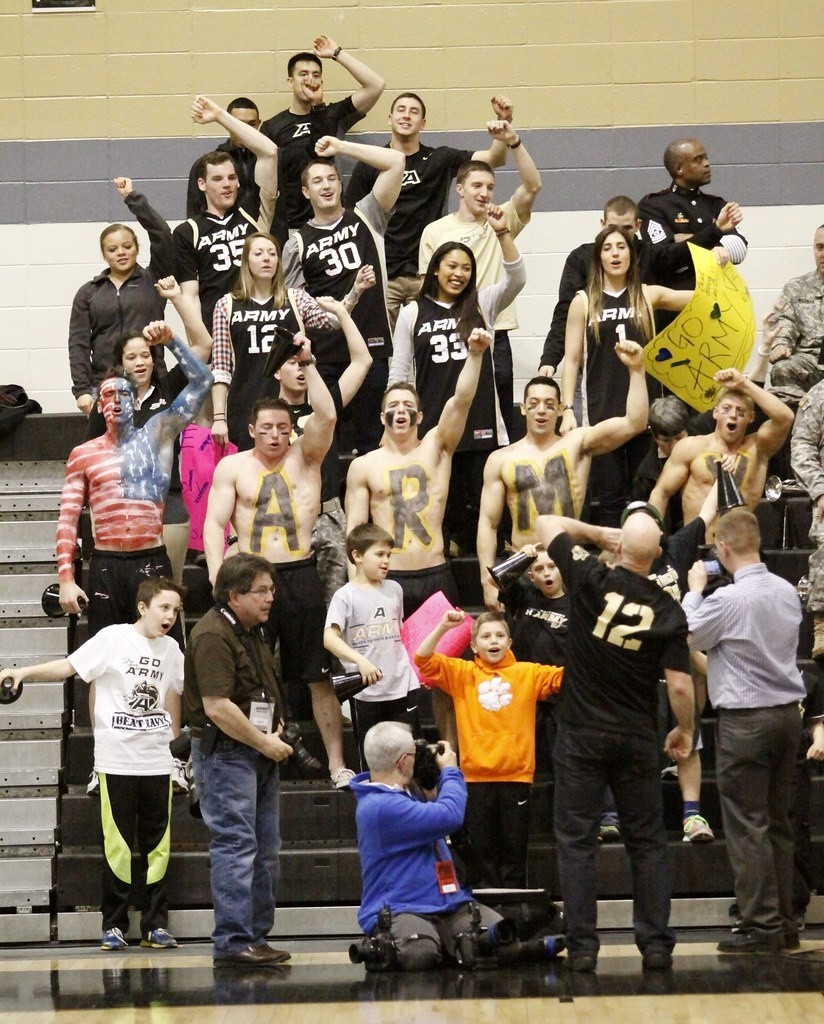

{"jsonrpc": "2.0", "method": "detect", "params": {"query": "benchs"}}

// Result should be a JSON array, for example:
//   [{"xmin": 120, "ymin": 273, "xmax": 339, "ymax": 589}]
[{"xmin": 0, "ymin": 404, "xmax": 824, "ymax": 940}]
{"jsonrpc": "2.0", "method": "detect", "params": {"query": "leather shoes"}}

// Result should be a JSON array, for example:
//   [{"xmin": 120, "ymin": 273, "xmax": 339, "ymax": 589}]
[
  {"xmin": 259, "ymin": 942, "xmax": 291, "ymax": 963},
  {"xmin": 213, "ymin": 945, "xmax": 287, "ymax": 966}
]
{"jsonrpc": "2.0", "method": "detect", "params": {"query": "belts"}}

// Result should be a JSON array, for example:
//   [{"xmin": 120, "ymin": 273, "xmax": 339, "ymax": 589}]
[
  {"xmin": 316, "ymin": 497, "xmax": 341, "ymax": 516},
  {"xmin": 190, "ymin": 728, "xmax": 205, "ymax": 739}
]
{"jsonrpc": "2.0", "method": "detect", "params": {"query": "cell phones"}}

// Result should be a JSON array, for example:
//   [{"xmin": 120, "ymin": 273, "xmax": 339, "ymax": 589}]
[{"xmin": 704, "ymin": 561, "xmax": 721, "ymax": 574}]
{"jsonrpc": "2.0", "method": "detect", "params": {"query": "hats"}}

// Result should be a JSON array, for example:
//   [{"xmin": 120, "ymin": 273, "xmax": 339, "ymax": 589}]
[{"xmin": 621, "ymin": 500, "xmax": 665, "ymax": 533}]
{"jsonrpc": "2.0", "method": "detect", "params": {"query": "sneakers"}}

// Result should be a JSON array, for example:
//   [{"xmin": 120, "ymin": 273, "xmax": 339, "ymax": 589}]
[
  {"xmin": 331, "ymin": 769, "xmax": 358, "ymax": 788},
  {"xmin": 598, "ymin": 825, "xmax": 620, "ymax": 842},
  {"xmin": 85, "ymin": 771, "xmax": 100, "ymax": 796},
  {"xmin": 170, "ymin": 758, "xmax": 189, "ymax": 795},
  {"xmin": 140, "ymin": 928, "xmax": 177, "ymax": 948},
  {"xmin": 100, "ymin": 926, "xmax": 129, "ymax": 950},
  {"xmin": 682, "ymin": 814, "xmax": 715, "ymax": 842}
]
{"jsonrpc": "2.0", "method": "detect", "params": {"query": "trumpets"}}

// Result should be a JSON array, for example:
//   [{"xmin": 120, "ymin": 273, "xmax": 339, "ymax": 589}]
[{"xmin": 764, "ymin": 474, "xmax": 809, "ymax": 503}]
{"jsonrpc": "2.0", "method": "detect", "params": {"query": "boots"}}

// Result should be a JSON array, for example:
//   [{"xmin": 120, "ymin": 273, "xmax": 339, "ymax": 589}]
[{"xmin": 810, "ymin": 612, "xmax": 824, "ymax": 660}]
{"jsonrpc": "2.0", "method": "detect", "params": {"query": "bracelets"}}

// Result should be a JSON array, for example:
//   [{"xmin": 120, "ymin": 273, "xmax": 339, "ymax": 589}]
[
  {"xmin": 494, "ymin": 228, "xmax": 510, "ymax": 236},
  {"xmin": 298, "ymin": 359, "xmax": 314, "ymax": 367},
  {"xmin": 757, "ymin": 346, "xmax": 771, "ymax": 357},
  {"xmin": 507, "ymin": 137, "xmax": 521, "ymax": 149},
  {"xmin": 332, "ymin": 47, "xmax": 342, "ymax": 61},
  {"xmin": 498, "ymin": 116, "xmax": 513, "ymax": 123},
  {"xmin": 344, "ymin": 295, "xmax": 358, "ymax": 305},
  {"xmin": 563, "ymin": 405, "xmax": 573, "ymax": 409}
]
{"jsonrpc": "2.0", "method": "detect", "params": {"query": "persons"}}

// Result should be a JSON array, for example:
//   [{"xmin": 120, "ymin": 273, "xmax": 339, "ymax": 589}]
[
  {"xmin": 327, "ymin": 501, "xmax": 698, "ymax": 974},
  {"xmin": 681, "ymin": 509, "xmax": 810, "ymax": 952},
  {"xmin": 68, "ymin": 34, "xmax": 824, "ymax": 589},
  {"xmin": 0, "ymin": 554, "xmax": 303, "ymax": 966},
  {"xmin": 55, "ymin": 319, "xmax": 824, "ymax": 787}
]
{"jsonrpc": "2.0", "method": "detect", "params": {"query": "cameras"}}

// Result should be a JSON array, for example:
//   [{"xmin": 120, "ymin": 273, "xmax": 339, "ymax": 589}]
[
  {"xmin": 261, "ymin": 721, "xmax": 322, "ymax": 780},
  {"xmin": 456, "ymin": 901, "xmax": 518, "ymax": 972},
  {"xmin": 411, "ymin": 738, "xmax": 444, "ymax": 783},
  {"xmin": 496, "ymin": 934, "xmax": 564, "ymax": 968},
  {"xmin": 348, "ymin": 908, "xmax": 397, "ymax": 972}
]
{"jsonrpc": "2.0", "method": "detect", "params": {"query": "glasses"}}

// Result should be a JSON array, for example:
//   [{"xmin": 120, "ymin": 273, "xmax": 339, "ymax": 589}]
[{"xmin": 246, "ymin": 586, "xmax": 277, "ymax": 598}]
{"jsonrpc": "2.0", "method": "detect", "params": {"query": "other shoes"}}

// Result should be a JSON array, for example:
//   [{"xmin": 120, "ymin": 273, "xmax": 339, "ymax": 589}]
[
  {"xmin": 784, "ymin": 929, "xmax": 800, "ymax": 949},
  {"xmin": 717, "ymin": 931, "xmax": 785, "ymax": 952},
  {"xmin": 791, "ymin": 914, "xmax": 804, "ymax": 932},
  {"xmin": 448, "ymin": 540, "xmax": 461, "ymax": 557},
  {"xmin": 731, "ymin": 918, "xmax": 743, "ymax": 932},
  {"xmin": 643, "ymin": 946, "xmax": 673, "ymax": 969},
  {"xmin": 341, "ymin": 714, "xmax": 353, "ymax": 729},
  {"xmin": 297, "ymin": 720, "xmax": 320, "ymax": 733},
  {"xmin": 572, "ymin": 954, "xmax": 597, "ymax": 972}
]
